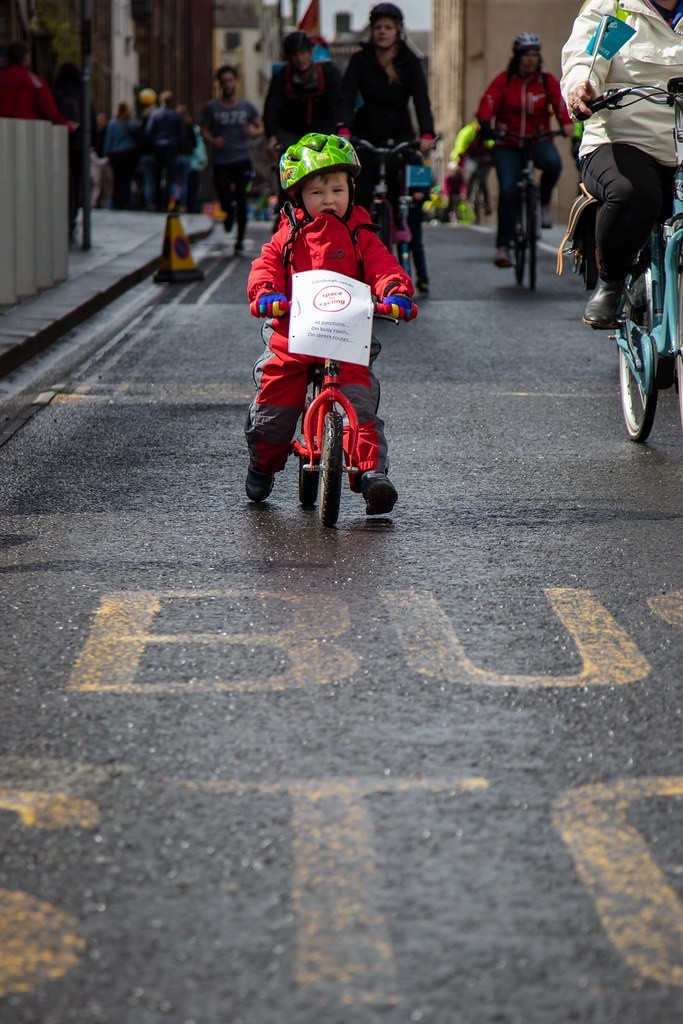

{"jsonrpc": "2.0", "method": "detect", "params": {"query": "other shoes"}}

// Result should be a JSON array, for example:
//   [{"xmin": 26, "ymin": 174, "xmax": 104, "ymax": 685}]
[
  {"xmin": 234, "ymin": 242, "xmax": 244, "ymax": 250},
  {"xmin": 493, "ymin": 247, "xmax": 511, "ymax": 270},
  {"xmin": 415, "ymin": 279, "xmax": 430, "ymax": 295},
  {"xmin": 540, "ymin": 209, "xmax": 553, "ymax": 227},
  {"xmin": 224, "ymin": 201, "xmax": 238, "ymax": 232}
]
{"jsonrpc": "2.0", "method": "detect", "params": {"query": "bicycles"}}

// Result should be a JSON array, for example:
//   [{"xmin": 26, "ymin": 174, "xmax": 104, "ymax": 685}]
[
  {"xmin": 342, "ymin": 134, "xmax": 444, "ymax": 292},
  {"xmin": 489, "ymin": 127, "xmax": 573, "ymax": 291},
  {"xmin": 247, "ymin": 289, "xmax": 418, "ymax": 528},
  {"xmin": 574, "ymin": 75, "xmax": 683, "ymax": 444},
  {"xmin": 456, "ymin": 155, "xmax": 493, "ymax": 227}
]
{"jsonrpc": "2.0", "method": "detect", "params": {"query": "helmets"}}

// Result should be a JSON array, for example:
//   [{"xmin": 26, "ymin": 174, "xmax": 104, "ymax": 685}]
[
  {"xmin": 370, "ymin": 3, "xmax": 404, "ymax": 21},
  {"xmin": 280, "ymin": 133, "xmax": 361, "ymax": 188},
  {"xmin": 280, "ymin": 31, "xmax": 314, "ymax": 60},
  {"xmin": 513, "ymin": 32, "xmax": 541, "ymax": 48}
]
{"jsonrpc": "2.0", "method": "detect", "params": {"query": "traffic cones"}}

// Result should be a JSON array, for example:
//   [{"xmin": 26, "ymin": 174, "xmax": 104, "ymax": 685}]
[{"xmin": 151, "ymin": 214, "xmax": 208, "ymax": 281}]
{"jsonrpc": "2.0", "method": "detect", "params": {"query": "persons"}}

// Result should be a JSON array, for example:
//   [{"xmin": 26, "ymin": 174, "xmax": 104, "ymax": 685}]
[
  {"xmin": 91, "ymin": 108, "xmax": 107, "ymax": 157},
  {"xmin": 422, "ymin": 110, "xmax": 491, "ymax": 225},
  {"xmin": 137, "ymin": 89, "xmax": 209, "ymax": 213},
  {"xmin": 0, "ymin": 41, "xmax": 77, "ymax": 132},
  {"xmin": 245, "ymin": 134, "xmax": 415, "ymax": 515},
  {"xmin": 201, "ymin": 66, "xmax": 264, "ymax": 254},
  {"xmin": 476, "ymin": 33, "xmax": 584, "ymax": 269},
  {"xmin": 261, "ymin": 32, "xmax": 344, "ymax": 235},
  {"xmin": 107, "ymin": 103, "xmax": 141, "ymax": 210},
  {"xmin": 333, "ymin": 3, "xmax": 437, "ymax": 295},
  {"xmin": 561, "ymin": 0, "xmax": 683, "ymax": 389},
  {"xmin": 52, "ymin": 61, "xmax": 95, "ymax": 244}
]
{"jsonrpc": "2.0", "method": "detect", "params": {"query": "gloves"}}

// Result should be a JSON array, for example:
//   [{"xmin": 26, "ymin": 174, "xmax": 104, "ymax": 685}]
[
  {"xmin": 259, "ymin": 295, "xmax": 287, "ymax": 318},
  {"xmin": 382, "ymin": 296, "xmax": 412, "ymax": 323}
]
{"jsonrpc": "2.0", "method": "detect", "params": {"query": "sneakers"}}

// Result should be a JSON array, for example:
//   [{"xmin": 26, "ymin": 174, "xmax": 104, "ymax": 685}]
[
  {"xmin": 365, "ymin": 479, "xmax": 398, "ymax": 515},
  {"xmin": 245, "ymin": 458, "xmax": 274, "ymax": 501}
]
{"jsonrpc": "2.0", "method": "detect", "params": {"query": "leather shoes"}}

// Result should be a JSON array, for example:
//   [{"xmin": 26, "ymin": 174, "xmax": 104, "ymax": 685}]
[{"xmin": 582, "ymin": 278, "xmax": 620, "ymax": 325}]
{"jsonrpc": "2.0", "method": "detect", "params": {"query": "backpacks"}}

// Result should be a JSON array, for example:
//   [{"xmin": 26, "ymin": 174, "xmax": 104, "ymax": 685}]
[{"xmin": 555, "ymin": 182, "xmax": 605, "ymax": 291}]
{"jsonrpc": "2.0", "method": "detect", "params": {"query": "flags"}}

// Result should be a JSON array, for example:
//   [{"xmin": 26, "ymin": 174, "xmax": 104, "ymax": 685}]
[{"xmin": 586, "ymin": 14, "xmax": 636, "ymax": 60}]
